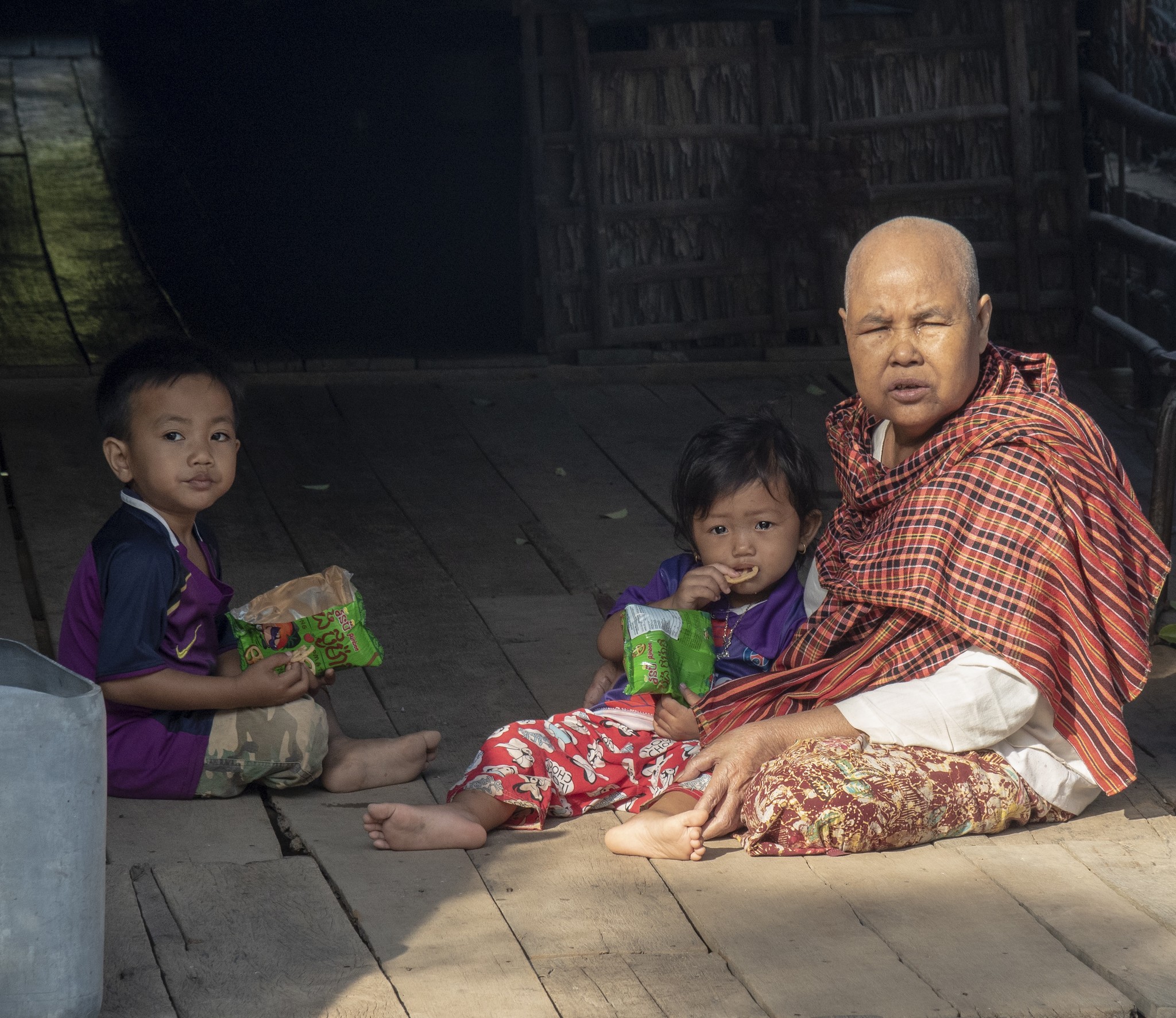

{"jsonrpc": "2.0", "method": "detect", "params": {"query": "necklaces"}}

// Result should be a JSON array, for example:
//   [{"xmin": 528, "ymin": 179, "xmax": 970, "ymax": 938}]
[{"xmin": 716, "ymin": 597, "xmax": 768, "ymax": 659}]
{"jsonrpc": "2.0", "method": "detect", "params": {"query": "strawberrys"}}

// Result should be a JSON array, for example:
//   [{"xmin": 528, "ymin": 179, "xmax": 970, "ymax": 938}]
[{"xmin": 304, "ymin": 632, "xmax": 315, "ymax": 643}]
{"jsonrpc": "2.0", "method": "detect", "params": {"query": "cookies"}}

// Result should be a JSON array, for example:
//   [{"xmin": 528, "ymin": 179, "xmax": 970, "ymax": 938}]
[
  {"xmin": 726, "ymin": 566, "xmax": 759, "ymax": 584},
  {"xmin": 290, "ymin": 646, "xmax": 315, "ymax": 662}
]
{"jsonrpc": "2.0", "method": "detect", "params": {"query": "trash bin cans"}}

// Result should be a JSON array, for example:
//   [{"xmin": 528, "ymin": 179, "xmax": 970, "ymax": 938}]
[{"xmin": 2, "ymin": 637, "xmax": 109, "ymax": 1017}]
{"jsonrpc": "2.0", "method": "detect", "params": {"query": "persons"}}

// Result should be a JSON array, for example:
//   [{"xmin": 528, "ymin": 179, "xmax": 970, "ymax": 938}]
[
  {"xmin": 362, "ymin": 406, "xmax": 824, "ymax": 862},
  {"xmin": 586, "ymin": 214, "xmax": 1173, "ymax": 857},
  {"xmin": 57, "ymin": 336, "xmax": 440, "ymax": 799}
]
